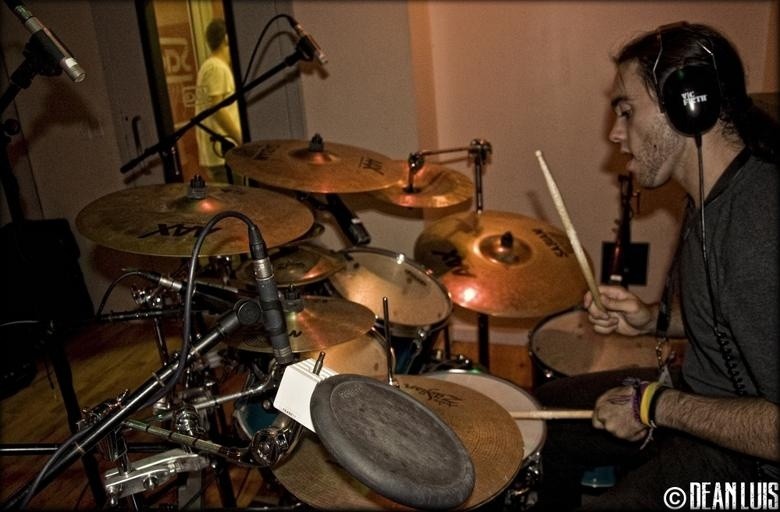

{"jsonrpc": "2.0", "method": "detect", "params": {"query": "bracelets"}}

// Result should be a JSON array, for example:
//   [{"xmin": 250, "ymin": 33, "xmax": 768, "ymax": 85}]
[{"xmin": 632, "ymin": 379, "xmax": 672, "ymax": 429}]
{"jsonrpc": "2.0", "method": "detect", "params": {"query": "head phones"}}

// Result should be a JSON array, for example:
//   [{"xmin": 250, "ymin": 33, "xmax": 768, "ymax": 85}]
[{"xmin": 655, "ymin": 21, "xmax": 722, "ymax": 137}]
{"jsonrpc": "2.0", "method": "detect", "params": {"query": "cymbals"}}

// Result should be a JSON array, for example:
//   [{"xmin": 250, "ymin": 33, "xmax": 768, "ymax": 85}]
[
  {"xmin": 415, "ymin": 208, "xmax": 595, "ymax": 318},
  {"xmin": 227, "ymin": 140, "xmax": 403, "ymax": 193},
  {"xmin": 370, "ymin": 159, "xmax": 474, "ymax": 208},
  {"xmin": 75, "ymin": 181, "xmax": 314, "ymax": 257},
  {"xmin": 220, "ymin": 294, "xmax": 375, "ymax": 355},
  {"xmin": 235, "ymin": 244, "xmax": 346, "ymax": 287},
  {"xmin": 271, "ymin": 374, "xmax": 524, "ymax": 511}
]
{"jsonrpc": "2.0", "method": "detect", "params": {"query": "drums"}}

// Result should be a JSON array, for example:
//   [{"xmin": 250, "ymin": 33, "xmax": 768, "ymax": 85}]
[
  {"xmin": 308, "ymin": 245, "xmax": 453, "ymax": 376},
  {"xmin": 529, "ymin": 306, "xmax": 670, "ymax": 491},
  {"xmin": 418, "ymin": 368, "xmax": 547, "ymax": 511},
  {"xmin": 230, "ymin": 326, "xmax": 397, "ymax": 445}
]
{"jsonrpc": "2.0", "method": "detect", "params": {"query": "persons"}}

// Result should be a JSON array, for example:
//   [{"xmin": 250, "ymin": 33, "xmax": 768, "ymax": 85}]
[
  {"xmin": 582, "ymin": 20, "xmax": 780, "ymax": 512},
  {"xmin": 195, "ymin": 18, "xmax": 244, "ymax": 185}
]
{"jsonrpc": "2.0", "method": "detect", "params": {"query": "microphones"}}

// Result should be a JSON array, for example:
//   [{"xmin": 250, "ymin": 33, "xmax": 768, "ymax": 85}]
[
  {"xmin": 247, "ymin": 222, "xmax": 295, "ymax": 364},
  {"xmin": 293, "ymin": 25, "xmax": 329, "ymax": 66},
  {"xmin": 5, "ymin": 0, "xmax": 88, "ymax": 85}
]
